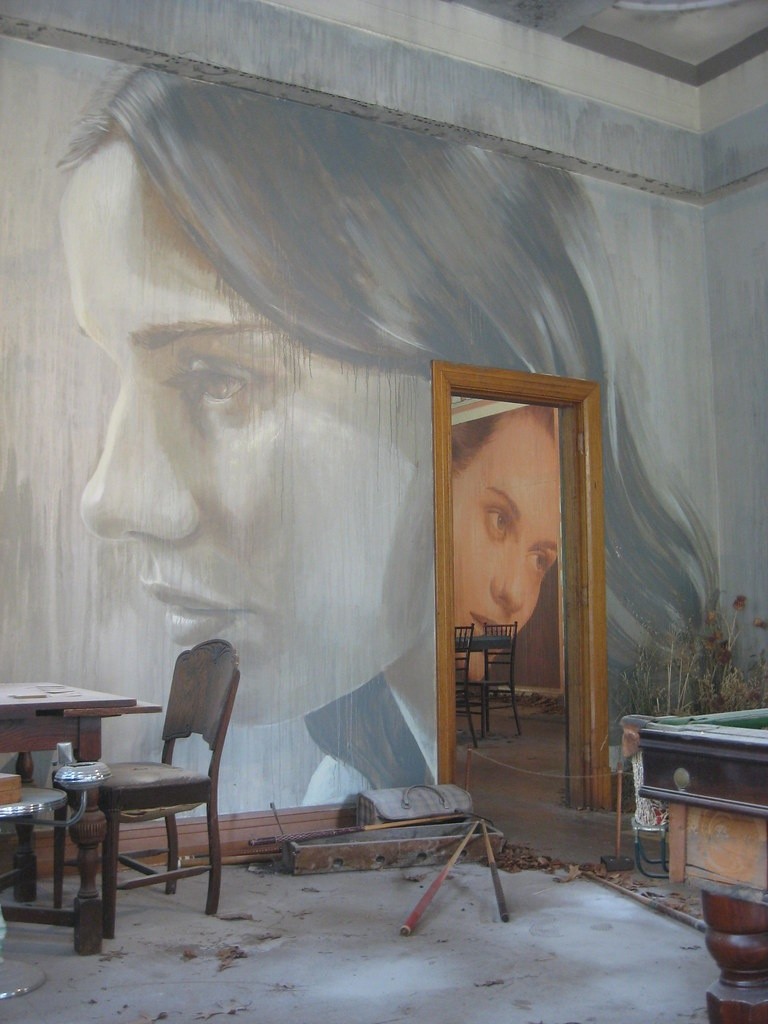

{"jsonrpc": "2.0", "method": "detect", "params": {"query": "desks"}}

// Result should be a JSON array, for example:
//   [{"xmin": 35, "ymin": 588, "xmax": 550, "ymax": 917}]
[
  {"xmin": 0, "ymin": 681, "xmax": 164, "ymax": 943},
  {"xmin": 636, "ymin": 706, "xmax": 768, "ymax": 1024},
  {"xmin": 454, "ymin": 633, "xmax": 511, "ymax": 651}
]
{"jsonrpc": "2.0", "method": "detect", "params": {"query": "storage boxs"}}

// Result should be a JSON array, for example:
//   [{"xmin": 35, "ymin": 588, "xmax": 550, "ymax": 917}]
[{"xmin": 0, "ymin": 773, "xmax": 22, "ymax": 805}]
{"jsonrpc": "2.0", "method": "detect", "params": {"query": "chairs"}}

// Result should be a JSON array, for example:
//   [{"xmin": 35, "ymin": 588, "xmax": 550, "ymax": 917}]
[
  {"xmin": 454, "ymin": 620, "xmax": 477, "ymax": 748},
  {"xmin": 469, "ymin": 620, "xmax": 522, "ymax": 737},
  {"xmin": 54, "ymin": 637, "xmax": 242, "ymax": 941}
]
